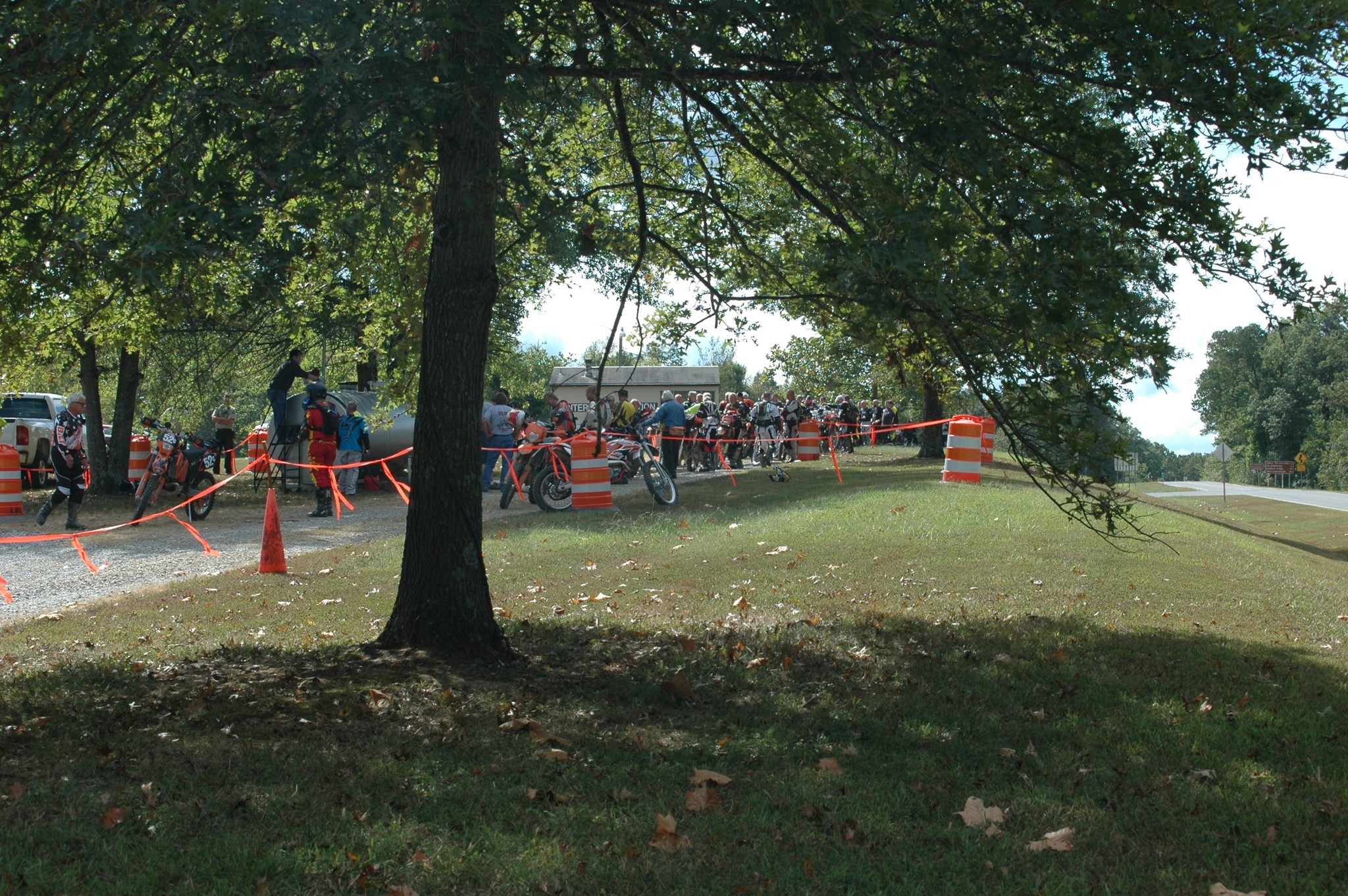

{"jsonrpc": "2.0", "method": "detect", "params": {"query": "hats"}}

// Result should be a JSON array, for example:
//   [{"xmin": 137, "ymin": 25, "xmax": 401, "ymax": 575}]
[
  {"xmin": 224, "ymin": 392, "xmax": 231, "ymax": 398},
  {"xmin": 737, "ymin": 392, "xmax": 743, "ymax": 397}
]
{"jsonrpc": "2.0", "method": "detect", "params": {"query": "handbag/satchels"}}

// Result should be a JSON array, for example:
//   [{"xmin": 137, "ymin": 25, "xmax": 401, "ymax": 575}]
[{"xmin": 669, "ymin": 425, "xmax": 685, "ymax": 436}]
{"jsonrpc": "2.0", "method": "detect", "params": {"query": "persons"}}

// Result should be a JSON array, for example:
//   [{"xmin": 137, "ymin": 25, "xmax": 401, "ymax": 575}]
[
  {"xmin": 606, "ymin": 389, "xmax": 641, "ymax": 429},
  {"xmin": 267, "ymin": 348, "xmax": 318, "ymax": 442},
  {"xmin": 719, "ymin": 391, "xmax": 755, "ymax": 470},
  {"xmin": 212, "ymin": 393, "xmax": 236, "ymax": 476},
  {"xmin": 637, "ymin": 390, "xmax": 686, "ymax": 479},
  {"xmin": 586, "ymin": 385, "xmax": 614, "ymax": 437},
  {"xmin": 294, "ymin": 383, "xmax": 341, "ymax": 517},
  {"xmin": 771, "ymin": 389, "xmax": 802, "ymax": 463},
  {"xmin": 857, "ymin": 399, "xmax": 898, "ymax": 446},
  {"xmin": 822, "ymin": 395, "xmax": 859, "ymax": 454},
  {"xmin": 904, "ymin": 419, "xmax": 918, "ymax": 447},
  {"xmin": 943, "ymin": 421, "xmax": 949, "ymax": 448},
  {"xmin": 681, "ymin": 391, "xmax": 721, "ymax": 471},
  {"xmin": 37, "ymin": 392, "xmax": 87, "ymax": 530},
  {"xmin": 544, "ymin": 393, "xmax": 575, "ymax": 441},
  {"xmin": 745, "ymin": 392, "xmax": 782, "ymax": 469},
  {"xmin": 479, "ymin": 388, "xmax": 516, "ymax": 492},
  {"xmin": 337, "ymin": 401, "xmax": 370, "ymax": 496},
  {"xmin": 1148, "ymin": 472, "xmax": 1152, "ymax": 482},
  {"xmin": 673, "ymin": 394, "xmax": 683, "ymax": 404},
  {"xmin": 795, "ymin": 394, "xmax": 826, "ymax": 454}
]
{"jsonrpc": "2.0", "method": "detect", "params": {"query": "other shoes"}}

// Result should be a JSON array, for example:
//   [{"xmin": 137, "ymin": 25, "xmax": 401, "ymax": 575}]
[
  {"xmin": 277, "ymin": 437, "xmax": 294, "ymax": 443},
  {"xmin": 735, "ymin": 463, "xmax": 744, "ymax": 468},
  {"xmin": 613, "ymin": 477, "xmax": 628, "ymax": 484},
  {"xmin": 763, "ymin": 454, "xmax": 771, "ymax": 466},
  {"xmin": 213, "ymin": 472, "xmax": 220, "ymax": 475},
  {"xmin": 671, "ymin": 474, "xmax": 677, "ymax": 479},
  {"xmin": 785, "ymin": 458, "xmax": 796, "ymax": 462},
  {"xmin": 730, "ymin": 461, "xmax": 736, "ymax": 468},
  {"xmin": 686, "ymin": 466, "xmax": 695, "ymax": 471},
  {"xmin": 481, "ymin": 486, "xmax": 490, "ymax": 491},
  {"xmin": 692, "ymin": 464, "xmax": 698, "ymax": 469},
  {"xmin": 838, "ymin": 448, "xmax": 854, "ymax": 454},
  {"xmin": 700, "ymin": 465, "xmax": 710, "ymax": 472},
  {"xmin": 225, "ymin": 472, "xmax": 232, "ymax": 475}
]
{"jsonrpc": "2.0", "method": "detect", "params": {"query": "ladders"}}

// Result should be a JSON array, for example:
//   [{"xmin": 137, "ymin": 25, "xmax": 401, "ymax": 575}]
[{"xmin": 253, "ymin": 388, "xmax": 301, "ymax": 492}]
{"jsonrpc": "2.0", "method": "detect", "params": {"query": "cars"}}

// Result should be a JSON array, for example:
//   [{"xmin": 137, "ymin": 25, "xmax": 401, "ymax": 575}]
[{"xmin": 102, "ymin": 424, "xmax": 135, "ymax": 448}]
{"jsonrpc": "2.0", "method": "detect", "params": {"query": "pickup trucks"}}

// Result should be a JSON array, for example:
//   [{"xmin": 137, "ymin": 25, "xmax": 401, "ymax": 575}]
[{"xmin": 0, "ymin": 392, "xmax": 89, "ymax": 490}]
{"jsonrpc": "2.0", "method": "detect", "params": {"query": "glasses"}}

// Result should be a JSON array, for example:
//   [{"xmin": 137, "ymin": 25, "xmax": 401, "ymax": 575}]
[
  {"xmin": 606, "ymin": 400, "xmax": 614, "ymax": 402},
  {"xmin": 72, "ymin": 401, "xmax": 85, "ymax": 407}
]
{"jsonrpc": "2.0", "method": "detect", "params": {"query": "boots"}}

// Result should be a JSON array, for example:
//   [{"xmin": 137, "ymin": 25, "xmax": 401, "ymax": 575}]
[
  {"xmin": 36, "ymin": 498, "xmax": 57, "ymax": 526},
  {"xmin": 307, "ymin": 488, "xmax": 333, "ymax": 517},
  {"xmin": 65, "ymin": 503, "xmax": 87, "ymax": 531}
]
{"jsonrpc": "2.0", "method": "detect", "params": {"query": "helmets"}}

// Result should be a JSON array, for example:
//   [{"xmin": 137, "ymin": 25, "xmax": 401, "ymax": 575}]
[
  {"xmin": 768, "ymin": 464, "xmax": 789, "ymax": 483},
  {"xmin": 304, "ymin": 381, "xmax": 327, "ymax": 401},
  {"xmin": 506, "ymin": 409, "xmax": 525, "ymax": 431}
]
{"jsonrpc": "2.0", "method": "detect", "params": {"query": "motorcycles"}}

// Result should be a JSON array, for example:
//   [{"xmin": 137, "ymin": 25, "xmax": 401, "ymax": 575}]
[
  {"xmin": 529, "ymin": 408, "xmax": 681, "ymax": 507},
  {"xmin": 497, "ymin": 414, "xmax": 568, "ymax": 509},
  {"xmin": 131, "ymin": 417, "xmax": 220, "ymax": 528},
  {"xmin": 640, "ymin": 406, "xmax": 847, "ymax": 472}
]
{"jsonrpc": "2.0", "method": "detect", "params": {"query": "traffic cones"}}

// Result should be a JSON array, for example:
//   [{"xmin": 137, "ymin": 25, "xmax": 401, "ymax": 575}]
[{"xmin": 259, "ymin": 491, "xmax": 290, "ymax": 573}]
